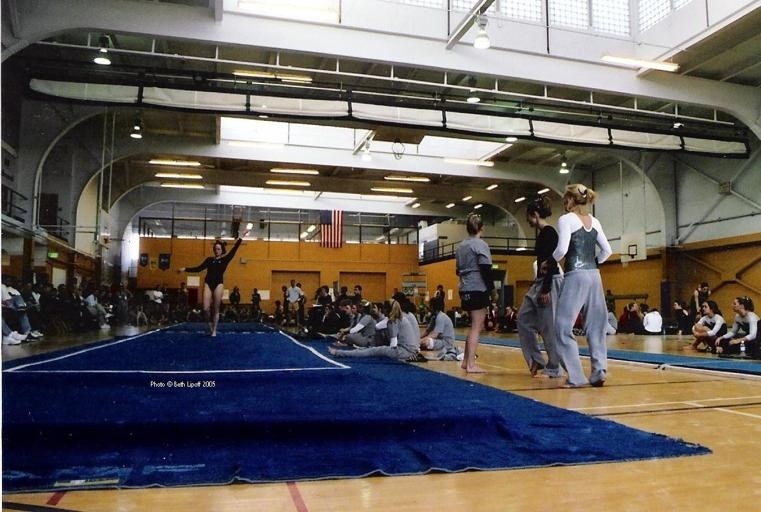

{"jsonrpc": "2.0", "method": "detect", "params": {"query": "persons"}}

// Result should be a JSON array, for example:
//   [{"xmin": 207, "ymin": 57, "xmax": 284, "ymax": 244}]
[
  {"xmin": 516, "ymin": 199, "xmax": 564, "ymax": 379},
  {"xmin": 455, "ymin": 213, "xmax": 499, "ymax": 372},
  {"xmin": 174, "ymin": 228, "xmax": 248, "ymax": 338},
  {"xmin": 2, "ymin": 276, "xmax": 761, "ymax": 361},
  {"xmin": 538, "ymin": 183, "xmax": 614, "ymax": 388}
]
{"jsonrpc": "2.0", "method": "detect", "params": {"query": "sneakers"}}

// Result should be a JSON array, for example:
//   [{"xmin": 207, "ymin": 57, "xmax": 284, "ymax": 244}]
[{"xmin": 3, "ymin": 330, "xmax": 43, "ymax": 345}]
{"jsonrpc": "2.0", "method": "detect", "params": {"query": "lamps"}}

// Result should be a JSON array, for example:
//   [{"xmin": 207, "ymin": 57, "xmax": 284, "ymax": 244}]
[
  {"xmin": 558, "ymin": 157, "xmax": 569, "ymax": 174},
  {"xmin": 465, "ymin": 84, "xmax": 481, "ymax": 103},
  {"xmin": 472, "ymin": 15, "xmax": 491, "ymax": 50},
  {"xmin": 93, "ymin": 38, "xmax": 111, "ymax": 64},
  {"xmin": 106, "ymin": 45, "xmax": 108, "ymax": 48},
  {"xmin": 362, "ymin": 140, "xmax": 373, "ymax": 162},
  {"xmin": 130, "ymin": 119, "xmax": 144, "ymax": 141}
]
